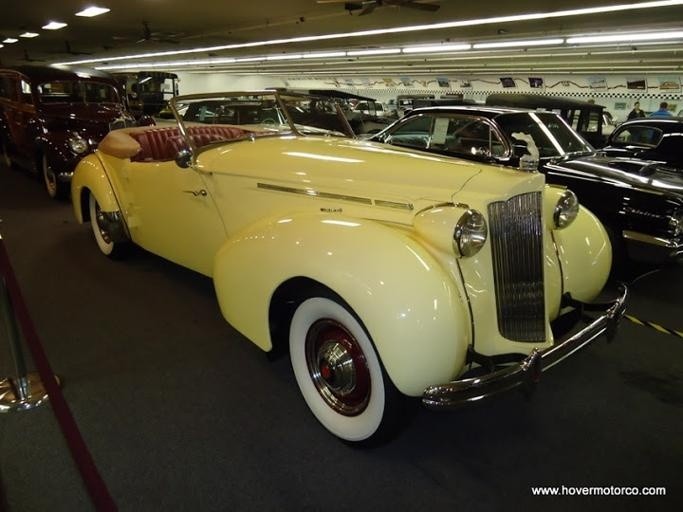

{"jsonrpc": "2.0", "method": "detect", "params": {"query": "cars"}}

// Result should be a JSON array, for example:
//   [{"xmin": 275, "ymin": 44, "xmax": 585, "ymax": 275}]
[{"xmin": 0, "ymin": 56, "xmax": 683, "ymax": 451}]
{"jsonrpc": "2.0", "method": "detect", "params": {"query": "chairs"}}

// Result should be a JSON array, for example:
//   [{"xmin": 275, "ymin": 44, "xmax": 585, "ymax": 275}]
[{"xmin": 130, "ymin": 125, "xmax": 261, "ymax": 162}]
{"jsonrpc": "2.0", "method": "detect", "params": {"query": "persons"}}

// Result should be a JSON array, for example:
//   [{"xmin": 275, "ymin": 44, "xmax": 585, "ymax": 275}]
[
  {"xmin": 626, "ymin": 101, "xmax": 646, "ymax": 121},
  {"xmin": 648, "ymin": 101, "xmax": 673, "ymax": 118}
]
